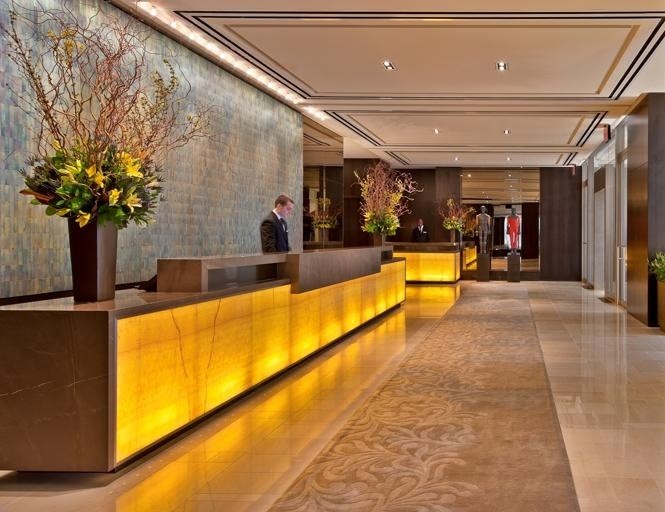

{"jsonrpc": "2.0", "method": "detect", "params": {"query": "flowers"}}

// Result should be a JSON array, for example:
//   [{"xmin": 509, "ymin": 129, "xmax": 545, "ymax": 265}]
[
  {"xmin": 353, "ymin": 158, "xmax": 426, "ymax": 235},
  {"xmin": 304, "ymin": 198, "xmax": 343, "ymax": 229},
  {"xmin": 1, "ymin": 0, "xmax": 162, "ymax": 229},
  {"xmin": 438, "ymin": 194, "xmax": 480, "ymax": 237}
]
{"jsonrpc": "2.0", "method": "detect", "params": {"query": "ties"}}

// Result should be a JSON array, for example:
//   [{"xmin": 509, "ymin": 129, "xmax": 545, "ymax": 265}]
[{"xmin": 420, "ymin": 227, "xmax": 421, "ymax": 232}]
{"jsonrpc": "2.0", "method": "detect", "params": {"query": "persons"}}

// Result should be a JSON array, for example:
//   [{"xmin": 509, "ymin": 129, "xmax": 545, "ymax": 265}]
[
  {"xmin": 505, "ymin": 208, "xmax": 521, "ymax": 256},
  {"xmin": 475, "ymin": 206, "xmax": 492, "ymax": 253},
  {"xmin": 303, "ymin": 206, "xmax": 316, "ymax": 241},
  {"xmin": 410, "ymin": 218, "xmax": 432, "ymax": 243},
  {"xmin": 260, "ymin": 194, "xmax": 295, "ymax": 252}
]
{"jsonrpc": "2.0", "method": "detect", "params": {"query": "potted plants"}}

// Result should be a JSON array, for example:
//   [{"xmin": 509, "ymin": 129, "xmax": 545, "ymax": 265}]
[{"xmin": 648, "ymin": 252, "xmax": 665, "ymax": 334}]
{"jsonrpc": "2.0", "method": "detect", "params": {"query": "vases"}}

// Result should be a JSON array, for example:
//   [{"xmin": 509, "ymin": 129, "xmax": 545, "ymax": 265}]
[
  {"xmin": 67, "ymin": 212, "xmax": 118, "ymax": 305},
  {"xmin": 373, "ymin": 233, "xmax": 386, "ymax": 248}
]
